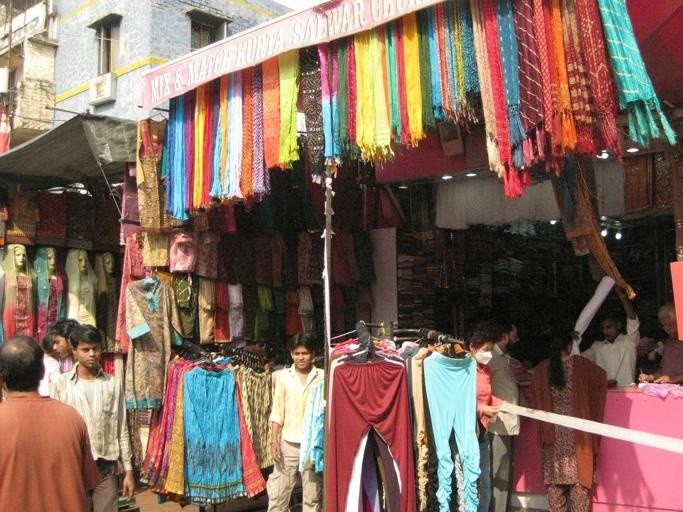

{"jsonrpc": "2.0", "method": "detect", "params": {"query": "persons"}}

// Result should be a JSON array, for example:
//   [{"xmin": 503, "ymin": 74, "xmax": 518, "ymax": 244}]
[
  {"xmin": 464, "ymin": 322, "xmax": 501, "ymax": 512},
  {"xmin": 266, "ymin": 332, "xmax": 324, "ymax": 512},
  {"xmin": 529, "ymin": 323, "xmax": 607, "ymax": 512},
  {"xmin": 486, "ymin": 320, "xmax": 520, "ymax": 511},
  {"xmin": 581, "ymin": 286, "xmax": 640, "ymax": 386},
  {"xmin": 0, "ymin": 318, "xmax": 136, "ymax": 512},
  {"xmin": 638, "ymin": 300, "xmax": 682, "ymax": 383}
]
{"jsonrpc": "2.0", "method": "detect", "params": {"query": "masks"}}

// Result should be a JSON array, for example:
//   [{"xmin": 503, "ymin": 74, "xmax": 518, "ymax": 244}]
[{"xmin": 472, "ymin": 346, "xmax": 493, "ymax": 365}]
